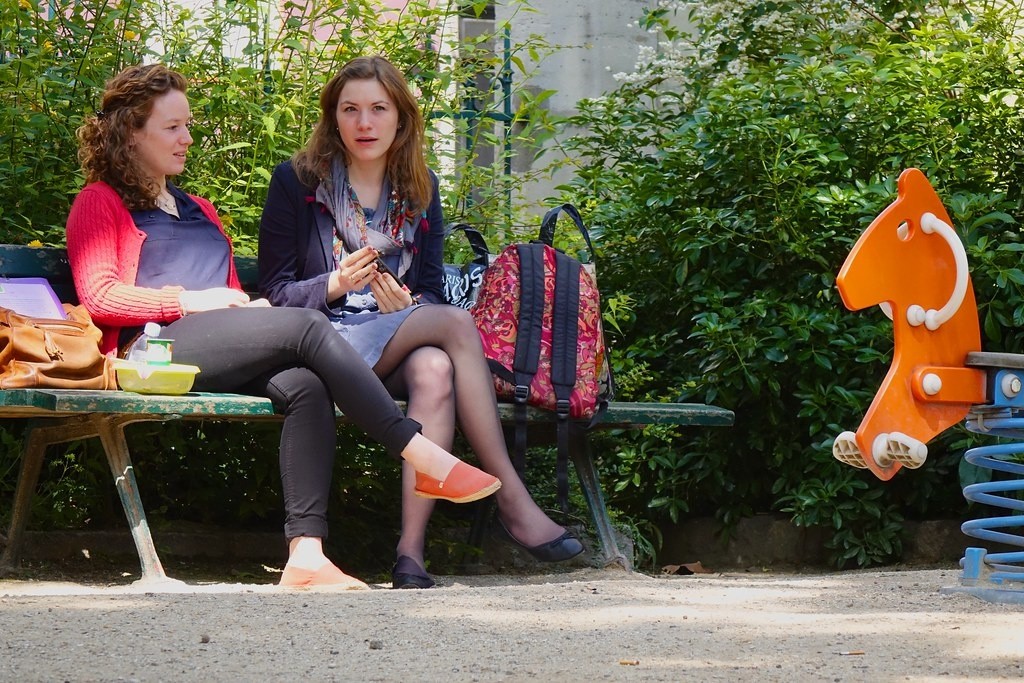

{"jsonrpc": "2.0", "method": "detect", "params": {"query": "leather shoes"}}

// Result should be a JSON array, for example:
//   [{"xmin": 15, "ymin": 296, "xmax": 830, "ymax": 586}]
[
  {"xmin": 392, "ymin": 565, "xmax": 424, "ymax": 589},
  {"xmin": 493, "ymin": 516, "xmax": 585, "ymax": 563}
]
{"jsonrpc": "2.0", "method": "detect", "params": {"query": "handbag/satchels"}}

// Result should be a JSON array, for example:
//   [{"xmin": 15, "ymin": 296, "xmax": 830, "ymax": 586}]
[
  {"xmin": 442, "ymin": 204, "xmax": 615, "ymax": 401},
  {"xmin": 0, "ymin": 303, "xmax": 119, "ymax": 390}
]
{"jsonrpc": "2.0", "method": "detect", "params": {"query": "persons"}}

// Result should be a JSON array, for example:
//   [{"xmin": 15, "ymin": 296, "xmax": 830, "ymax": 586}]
[
  {"xmin": 259, "ymin": 55, "xmax": 585, "ymax": 588},
  {"xmin": 66, "ymin": 64, "xmax": 502, "ymax": 586}
]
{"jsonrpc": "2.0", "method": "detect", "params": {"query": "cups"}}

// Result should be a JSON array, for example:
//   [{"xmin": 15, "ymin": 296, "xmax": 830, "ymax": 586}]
[{"xmin": 146, "ymin": 338, "xmax": 175, "ymax": 366}]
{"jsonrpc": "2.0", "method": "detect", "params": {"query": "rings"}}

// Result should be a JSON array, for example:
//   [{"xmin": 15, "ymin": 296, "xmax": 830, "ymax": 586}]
[{"xmin": 350, "ymin": 276, "xmax": 356, "ymax": 283}]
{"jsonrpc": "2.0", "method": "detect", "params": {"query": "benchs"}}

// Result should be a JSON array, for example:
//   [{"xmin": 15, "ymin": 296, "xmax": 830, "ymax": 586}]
[{"xmin": 0, "ymin": 246, "xmax": 738, "ymax": 593}]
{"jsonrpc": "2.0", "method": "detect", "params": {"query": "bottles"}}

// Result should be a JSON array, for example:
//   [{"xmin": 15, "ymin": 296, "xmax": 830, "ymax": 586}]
[{"xmin": 126, "ymin": 322, "xmax": 162, "ymax": 364}]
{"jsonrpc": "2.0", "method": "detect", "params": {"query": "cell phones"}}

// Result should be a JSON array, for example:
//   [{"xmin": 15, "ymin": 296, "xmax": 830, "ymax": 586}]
[{"xmin": 364, "ymin": 256, "xmax": 403, "ymax": 289}]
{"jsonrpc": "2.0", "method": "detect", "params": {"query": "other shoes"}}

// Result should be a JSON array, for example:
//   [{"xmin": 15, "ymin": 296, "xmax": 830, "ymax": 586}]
[
  {"xmin": 413, "ymin": 461, "xmax": 502, "ymax": 503},
  {"xmin": 279, "ymin": 562, "xmax": 368, "ymax": 589}
]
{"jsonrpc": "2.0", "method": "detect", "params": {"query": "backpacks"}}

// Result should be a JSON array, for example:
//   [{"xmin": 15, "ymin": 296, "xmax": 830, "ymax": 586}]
[{"xmin": 469, "ymin": 239, "xmax": 604, "ymax": 419}]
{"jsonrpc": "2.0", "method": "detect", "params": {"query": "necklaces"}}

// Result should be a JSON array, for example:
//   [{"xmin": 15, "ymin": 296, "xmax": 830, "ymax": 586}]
[{"xmin": 159, "ymin": 199, "xmax": 176, "ymax": 210}]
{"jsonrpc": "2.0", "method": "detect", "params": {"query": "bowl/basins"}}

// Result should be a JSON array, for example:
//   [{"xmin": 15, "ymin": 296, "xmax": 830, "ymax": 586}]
[{"xmin": 114, "ymin": 363, "xmax": 201, "ymax": 394}]
